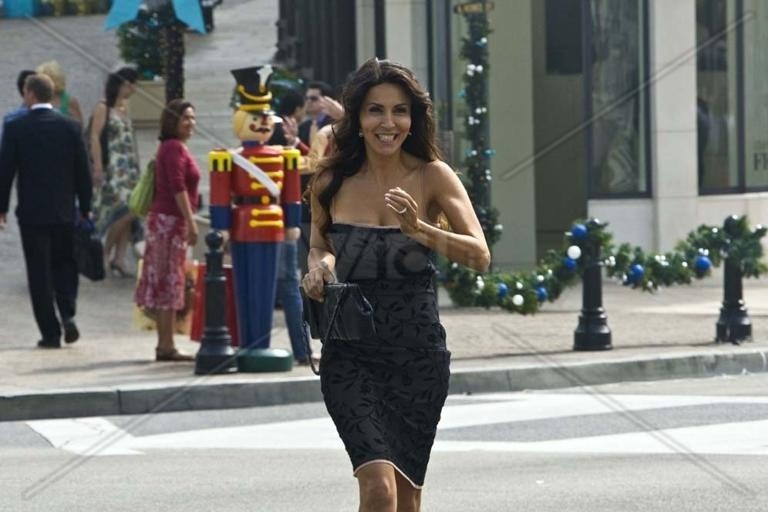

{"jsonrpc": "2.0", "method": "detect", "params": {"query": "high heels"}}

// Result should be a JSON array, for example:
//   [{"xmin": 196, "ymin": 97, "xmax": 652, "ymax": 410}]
[{"xmin": 109, "ymin": 259, "xmax": 135, "ymax": 278}]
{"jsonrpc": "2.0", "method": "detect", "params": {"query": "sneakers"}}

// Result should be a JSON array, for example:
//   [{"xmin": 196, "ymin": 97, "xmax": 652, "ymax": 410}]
[
  {"xmin": 38, "ymin": 337, "xmax": 61, "ymax": 348},
  {"xmin": 64, "ymin": 320, "xmax": 78, "ymax": 344}
]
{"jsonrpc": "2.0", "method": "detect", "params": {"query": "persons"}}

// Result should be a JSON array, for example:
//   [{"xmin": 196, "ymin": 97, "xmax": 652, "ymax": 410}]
[
  {"xmin": 2, "ymin": 71, "xmax": 40, "ymax": 122},
  {"xmin": 303, "ymin": 56, "xmax": 492, "ymax": 512},
  {"xmin": 87, "ymin": 66, "xmax": 141, "ymax": 280},
  {"xmin": 272, "ymin": 82, "xmax": 313, "ymax": 366},
  {"xmin": 40, "ymin": 59, "xmax": 81, "ymax": 116},
  {"xmin": 0, "ymin": 74, "xmax": 92, "ymax": 349},
  {"xmin": 202, "ymin": 61, "xmax": 300, "ymax": 352},
  {"xmin": 133, "ymin": 100, "xmax": 200, "ymax": 367},
  {"xmin": 292, "ymin": 81, "xmax": 342, "ymax": 145}
]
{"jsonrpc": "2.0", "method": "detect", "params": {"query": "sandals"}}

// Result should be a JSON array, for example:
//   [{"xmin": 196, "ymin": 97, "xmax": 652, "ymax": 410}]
[{"xmin": 157, "ymin": 348, "xmax": 195, "ymax": 360}]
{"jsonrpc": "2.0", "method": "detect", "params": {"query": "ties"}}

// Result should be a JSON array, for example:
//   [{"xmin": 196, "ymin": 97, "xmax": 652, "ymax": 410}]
[{"xmin": 309, "ymin": 120, "xmax": 319, "ymax": 146}]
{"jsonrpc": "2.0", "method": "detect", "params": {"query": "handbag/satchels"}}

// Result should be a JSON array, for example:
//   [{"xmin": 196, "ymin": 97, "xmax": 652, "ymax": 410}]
[
  {"xmin": 59, "ymin": 215, "xmax": 104, "ymax": 282},
  {"xmin": 83, "ymin": 126, "xmax": 109, "ymax": 168},
  {"xmin": 296, "ymin": 282, "xmax": 379, "ymax": 343},
  {"xmin": 127, "ymin": 158, "xmax": 156, "ymax": 219}
]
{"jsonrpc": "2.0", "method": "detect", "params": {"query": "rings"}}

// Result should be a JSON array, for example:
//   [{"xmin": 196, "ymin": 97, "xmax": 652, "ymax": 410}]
[{"xmin": 399, "ymin": 207, "xmax": 408, "ymax": 213}]
